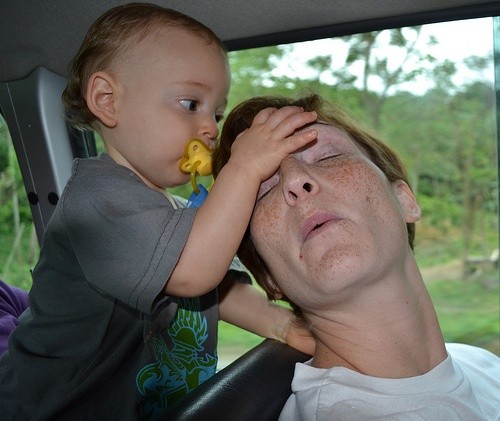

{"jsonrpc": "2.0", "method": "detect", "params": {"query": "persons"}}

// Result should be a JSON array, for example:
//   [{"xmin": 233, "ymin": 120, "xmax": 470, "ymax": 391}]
[
  {"xmin": 0, "ymin": 2, "xmax": 318, "ymax": 421},
  {"xmin": 210, "ymin": 93, "xmax": 500, "ymax": 421}
]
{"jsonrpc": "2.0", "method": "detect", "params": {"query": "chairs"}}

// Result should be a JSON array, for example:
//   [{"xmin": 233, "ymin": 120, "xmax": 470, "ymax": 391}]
[{"xmin": 161, "ymin": 338, "xmax": 313, "ymax": 421}]
